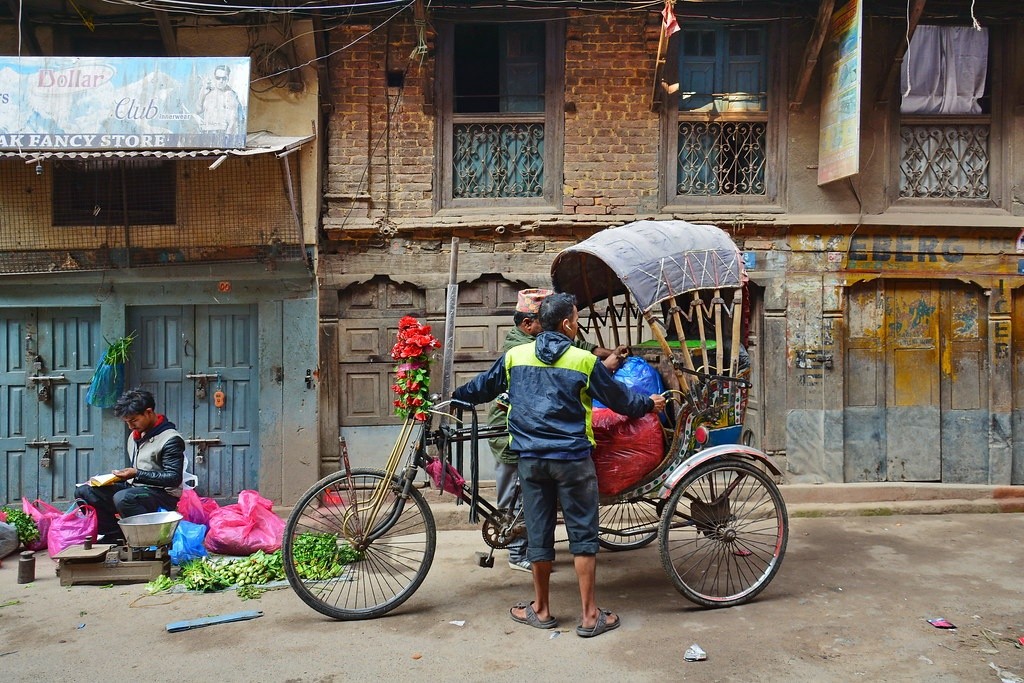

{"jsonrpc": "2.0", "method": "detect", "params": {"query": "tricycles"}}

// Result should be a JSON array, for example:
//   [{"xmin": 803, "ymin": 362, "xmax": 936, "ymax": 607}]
[{"xmin": 278, "ymin": 217, "xmax": 789, "ymax": 622}]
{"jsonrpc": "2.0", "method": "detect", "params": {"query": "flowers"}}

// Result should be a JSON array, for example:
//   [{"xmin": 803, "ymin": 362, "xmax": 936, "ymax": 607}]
[{"xmin": 389, "ymin": 316, "xmax": 441, "ymax": 422}]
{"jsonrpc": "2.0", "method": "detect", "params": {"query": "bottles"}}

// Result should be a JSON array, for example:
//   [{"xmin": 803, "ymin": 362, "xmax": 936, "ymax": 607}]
[
  {"xmin": 18, "ymin": 550, "xmax": 35, "ymax": 584},
  {"xmin": 85, "ymin": 536, "xmax": 92, "ymax": 550}
]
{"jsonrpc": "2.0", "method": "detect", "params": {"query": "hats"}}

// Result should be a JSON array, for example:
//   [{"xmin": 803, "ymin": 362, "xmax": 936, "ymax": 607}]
[{"xmin": 516, "ymin": 288, "xmax": 556, "ymax": 314}]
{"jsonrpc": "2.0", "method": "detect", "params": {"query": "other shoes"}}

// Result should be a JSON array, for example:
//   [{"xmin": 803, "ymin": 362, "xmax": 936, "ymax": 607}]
[
  {"xmin": 508, "ymin": 555, "xmax": 532, "ymax": 572},
  {"xmin": 97, "ymin": 529, "xmax": 125, "ymax": 544}
]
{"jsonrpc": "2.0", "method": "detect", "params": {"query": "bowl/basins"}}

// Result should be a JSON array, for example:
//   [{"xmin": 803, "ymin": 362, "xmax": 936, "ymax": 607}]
[{"xmin": 117, "ymin": 512, "xmax": 183, "ymax": 548}]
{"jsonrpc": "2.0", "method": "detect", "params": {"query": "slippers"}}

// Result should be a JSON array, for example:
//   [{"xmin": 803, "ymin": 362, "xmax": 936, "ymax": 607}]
[
  {"xmin": 509, "ymin": 603, "xmax": 558, "ymax": 628},
  {"xmin": 576, "ymin": 605, "xmax": 620, "ymax": 637}
]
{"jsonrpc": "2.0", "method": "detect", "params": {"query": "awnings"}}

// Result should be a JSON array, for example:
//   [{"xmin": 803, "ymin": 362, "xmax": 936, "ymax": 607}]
[{"xmin": 0, "ymin": 135, "xmax": 318, "ymax": 171}]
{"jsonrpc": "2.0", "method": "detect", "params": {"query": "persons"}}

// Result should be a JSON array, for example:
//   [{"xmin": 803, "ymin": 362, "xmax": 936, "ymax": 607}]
[
  {"xmin": 486, "ymin": 289, "xmax": 632, "ymax": 573},
  {"xmin": 74, "ymin": 389, "xmax": 185, "ymax": 547},
  {"xmin": 196, "ymin": 65, "xmax": 244, "ymax": 136},
  {"xmin": 450, "ymin": 293, "xmax": 665, "ymax": 637}
]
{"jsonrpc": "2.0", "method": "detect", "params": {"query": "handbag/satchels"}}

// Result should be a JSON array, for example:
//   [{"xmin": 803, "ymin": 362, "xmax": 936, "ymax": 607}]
[
  {"xmin": 157, "ymin": 487, "xmax": 289, "ymax": 568},
  {"xmin": 48, "ymin": 499, "xmax": 97, "ymax": 561},
  {"xmin": 19, "ymin": 494, "xmax": 62, "ymax": 552}
]
